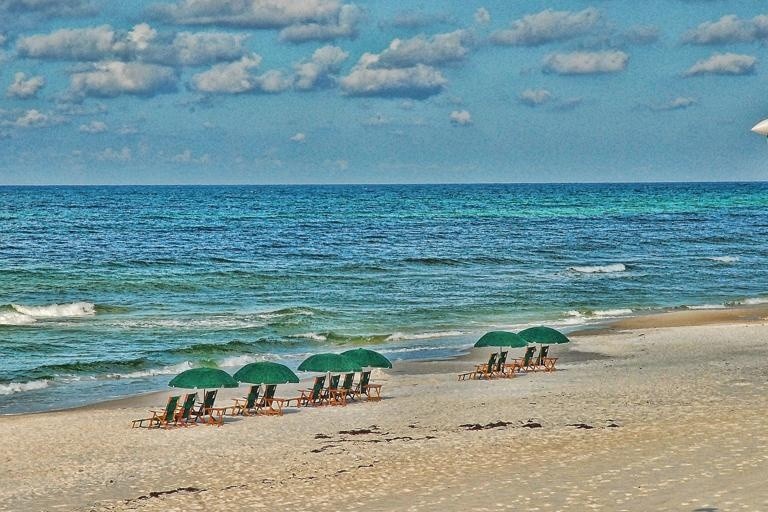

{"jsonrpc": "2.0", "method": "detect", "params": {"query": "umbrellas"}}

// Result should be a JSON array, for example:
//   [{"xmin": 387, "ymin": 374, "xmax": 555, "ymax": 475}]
[
  {"xmin": 474, "ymin": 331, "xmax": 529, "ymax": 353},
  {"xmin": 168, "ymin": 367, "xmax": 239, "ymax": 420},
  {"xmin": 517, "ymin": 327, "xmax": 570, "ymax": 368},
  {"xmin": 339, "ymin": 348, "xmax": 392, "ymax": 399},
  {"xmin": 233, "ymin": 361, "xmax": 300, "ymax": 415},
  {"xmin": 298, "ymin": 353, "xmax": 362, "ymax": 405}
]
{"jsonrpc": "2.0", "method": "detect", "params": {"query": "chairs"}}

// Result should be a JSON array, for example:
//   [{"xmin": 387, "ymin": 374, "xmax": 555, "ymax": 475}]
[
  {"xmin": 131, "ymin": 369, "xmax": 382, "ymax": 431},
  {"xmin": 457, "ymin": 344, "xmax": 558, "ymax": 381}
]
